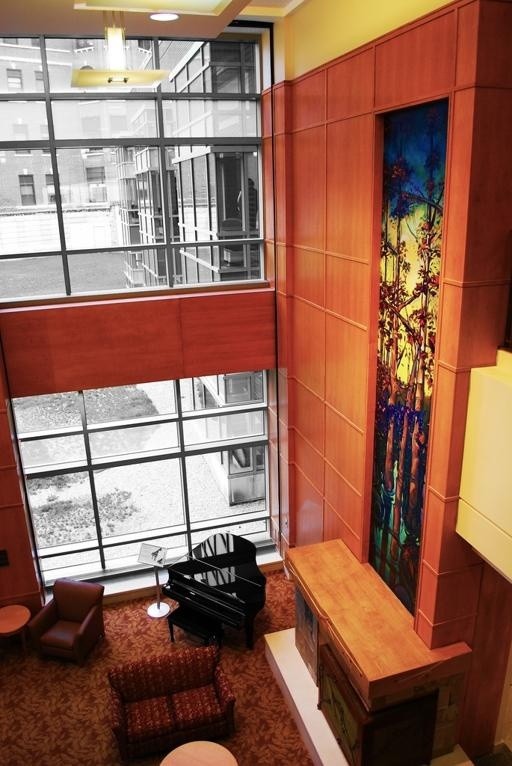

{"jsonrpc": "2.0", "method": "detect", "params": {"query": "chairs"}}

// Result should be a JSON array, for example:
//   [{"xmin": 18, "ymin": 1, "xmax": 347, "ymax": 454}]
[{"xmin": 29, "ymin": 578, "xmax": 108, "ymax": 671}]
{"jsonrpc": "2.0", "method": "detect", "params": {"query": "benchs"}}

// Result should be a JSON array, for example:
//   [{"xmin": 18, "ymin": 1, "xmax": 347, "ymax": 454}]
[{"xmin": 104, "ymin": 638, "xmax": 238, "ymax": 761}]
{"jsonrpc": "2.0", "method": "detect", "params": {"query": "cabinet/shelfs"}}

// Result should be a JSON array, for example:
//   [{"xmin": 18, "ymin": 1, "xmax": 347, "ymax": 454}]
[{"xmin": 316, "ymin": 642, "xmax": 441, "ymax": 766}]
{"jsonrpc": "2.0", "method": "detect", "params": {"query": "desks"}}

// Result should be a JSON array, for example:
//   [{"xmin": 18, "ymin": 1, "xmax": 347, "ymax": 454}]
[
  {"xmin": 0, "ymin": 603, "xmax": 33, "ymax": 663},
  {"xmin": 158, "ymin": 740, "xmax": 240, "ymax": 766}
]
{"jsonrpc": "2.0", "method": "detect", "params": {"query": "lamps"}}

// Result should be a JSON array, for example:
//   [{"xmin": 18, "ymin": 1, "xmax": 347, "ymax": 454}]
[{"xmin": 68, "ymin": 8, "xmax": 169, "ymax": 91}]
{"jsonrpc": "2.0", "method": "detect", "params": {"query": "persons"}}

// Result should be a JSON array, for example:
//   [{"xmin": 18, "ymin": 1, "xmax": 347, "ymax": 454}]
[{"xmin": 237, "ymin": 178, "xmax": 258, "ymax": 231}]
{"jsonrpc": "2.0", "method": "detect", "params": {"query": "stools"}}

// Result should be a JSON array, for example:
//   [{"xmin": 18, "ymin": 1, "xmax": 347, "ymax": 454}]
[{"xmin": 166, "ymin": 605, "xmax": 227, "ymax": 651}]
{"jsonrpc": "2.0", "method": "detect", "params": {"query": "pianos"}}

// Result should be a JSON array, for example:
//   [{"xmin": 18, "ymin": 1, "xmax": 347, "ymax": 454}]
[{"xmin": 162, "ymin": 532, "xmax": 266, "ymax": 650}]
